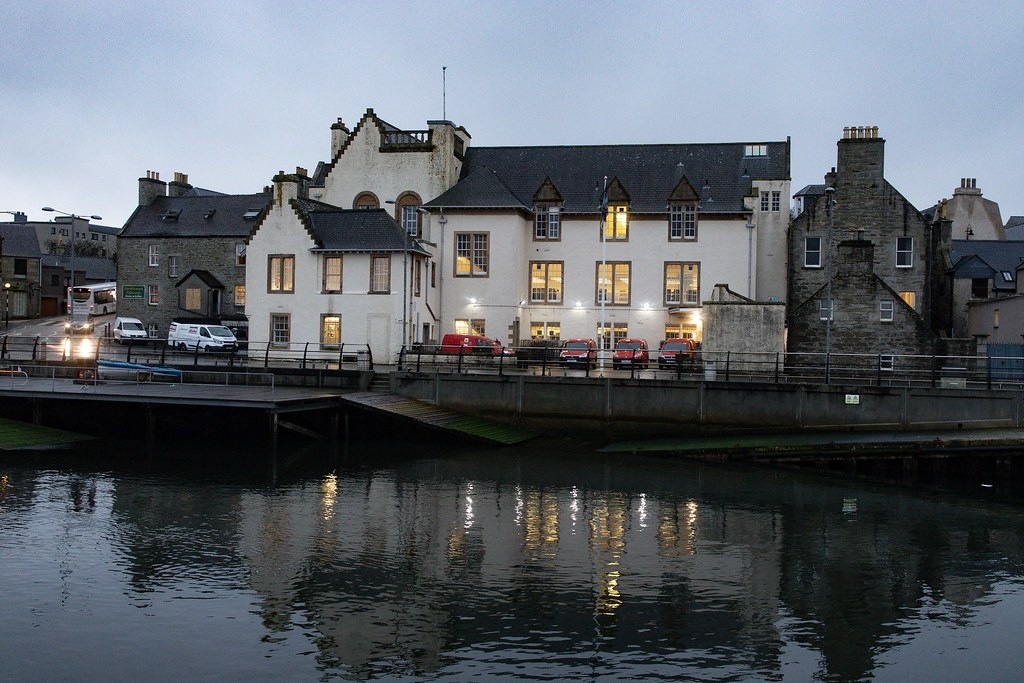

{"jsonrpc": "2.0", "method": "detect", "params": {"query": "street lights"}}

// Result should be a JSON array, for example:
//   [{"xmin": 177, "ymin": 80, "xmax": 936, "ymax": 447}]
[
  {"xmin": 822, "ymin": 185, "xmax": 837, "ymax": 380},
  {"xmin": 385, "ymin": 199, "xmax": 426, "ymax": 371},
  {"xmin": 5, "ymin": 282, "xmax": 11, "ymax": 326},
  {"xmin": 41, "ymin": 206, "xmax": 103, "ymax": 364}
]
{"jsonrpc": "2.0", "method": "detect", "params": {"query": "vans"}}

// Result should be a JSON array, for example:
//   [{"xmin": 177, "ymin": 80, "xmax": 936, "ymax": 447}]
[
  {"xmin": 165, "ymin": 321, "xmax": 240, "ymax": 353},
  {"xmin": 114, "ymin": 316, "xmax": 148, "ymax": 346},
  {"xmin": 439, "ymin": 332, "xmax": 513, "ymax": 356}
]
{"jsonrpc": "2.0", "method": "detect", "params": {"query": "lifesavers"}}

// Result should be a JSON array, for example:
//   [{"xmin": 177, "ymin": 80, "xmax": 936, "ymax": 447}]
[{"xmin": 79, "ymin": 368, "xmax": 95, "ymax": 379}]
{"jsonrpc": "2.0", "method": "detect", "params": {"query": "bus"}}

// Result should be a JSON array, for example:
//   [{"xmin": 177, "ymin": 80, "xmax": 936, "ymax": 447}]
[{"xmin": 67, "ymin": 280, "xmax": 117, "ymax": 317}]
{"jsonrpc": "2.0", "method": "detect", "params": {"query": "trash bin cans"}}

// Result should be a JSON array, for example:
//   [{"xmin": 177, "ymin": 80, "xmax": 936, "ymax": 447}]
[
  {"xmin": 357, "ymin": 350, "xmax": 371, "ymax": 370},
  {"xmin": 704, "ymin": 359, "xmax": 717, "ymax": 381},
  {"xmin": 342, "ymin": 354, "xmax": 357, "ymax": 370}
]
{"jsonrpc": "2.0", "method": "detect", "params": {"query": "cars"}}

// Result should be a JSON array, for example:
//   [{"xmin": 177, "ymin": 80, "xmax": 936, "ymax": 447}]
[
  {"xmin": 613, "ymin": 338, "xmax": 650, "ymax": 370},
  {"xmin": 560, "ymin": 338, "xmax": 599, "ymax": 370},
  {"xmin": 658, "ymin": 337, "xmax": 696, "ymax": 370},
  {"xmin": 65, "ymin": 313, "xmax": 95, "ymax": 335}
]
